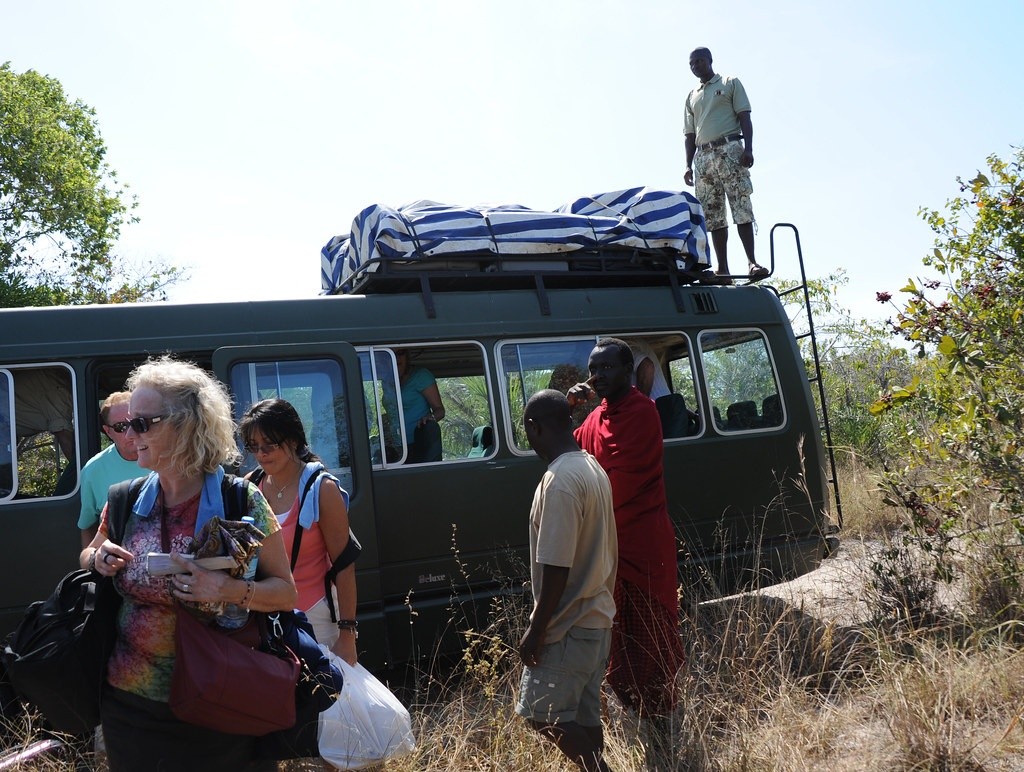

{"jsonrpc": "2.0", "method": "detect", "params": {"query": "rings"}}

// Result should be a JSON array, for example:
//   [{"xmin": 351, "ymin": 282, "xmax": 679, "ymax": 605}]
[
  {"xmin": 183, "ymin": 584, "xmax": 188, "ymax": 592},
  {"xmin": 104, "ymin": 554, "xmax": 109, "ymax": 563}
]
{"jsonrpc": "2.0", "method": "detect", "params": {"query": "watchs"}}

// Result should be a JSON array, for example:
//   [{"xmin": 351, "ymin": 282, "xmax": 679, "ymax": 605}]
[{"xmin": 88, "ymin": 548, "xmax": 97, "ymax": 573}]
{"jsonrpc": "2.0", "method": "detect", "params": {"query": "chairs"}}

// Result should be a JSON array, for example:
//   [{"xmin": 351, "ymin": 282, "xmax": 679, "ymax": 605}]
[
  {"xmin": 468, "ymin": 426, "xmax": 493, "ymax": 460},
  {"xmin": 405, "ymin": 418, "xmax": 441, "ymax": 464},
  {"xmin": 654, "ymin": 392, "xmax": 781, "ymax": 438}
]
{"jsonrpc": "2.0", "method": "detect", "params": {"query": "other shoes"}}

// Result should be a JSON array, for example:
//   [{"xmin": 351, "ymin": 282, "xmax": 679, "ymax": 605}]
[
  {"xmin": 749, "ymin": 264, "xmax": 768, "ymax": 281},
  {"xmin": 699, "ymin": 273, "xmax": 732, "ymax": 285}
]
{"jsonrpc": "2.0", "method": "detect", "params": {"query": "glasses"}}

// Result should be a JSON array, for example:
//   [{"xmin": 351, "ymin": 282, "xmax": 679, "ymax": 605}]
[
  {"xmin": 244, "ymin": 436, "xmax": 287, "ymax": 453},
  {"xmin": 125, "ymin": 414, "xmax": 175, "ymax": 433},
  {"xmin": 107, "ymin": 422, "xmax": 128, "ymax": 433}
]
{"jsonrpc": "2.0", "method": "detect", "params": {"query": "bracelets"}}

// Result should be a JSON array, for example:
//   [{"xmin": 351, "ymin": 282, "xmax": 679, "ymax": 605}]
[
  {"xmin": 430, "ymin": 413, "xmax": 436, "ymax": 420},
  {"xmin": 337, "ymin": 619, "xmax": 359, "ymax": 635},
  {"xmin": 235, "ymin": 579, "xmax": 256, "ymax": 612}
]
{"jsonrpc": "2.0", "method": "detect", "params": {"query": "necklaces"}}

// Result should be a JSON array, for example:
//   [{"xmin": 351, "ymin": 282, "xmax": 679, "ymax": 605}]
[{"xmin": 273, "ymin": 463, "xmax": 306, "ymax": 499}]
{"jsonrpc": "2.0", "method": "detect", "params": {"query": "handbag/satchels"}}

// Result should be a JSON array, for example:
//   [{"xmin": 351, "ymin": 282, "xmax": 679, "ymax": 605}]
[
  {"xmin": 318, "ymin": 643, "xmax": 418, "ymax": 770},
  {"xmin": 0, "ymin": 479, "xmax": 133, "ymax": 735},
  {"xmin": 169, "ymin": 606, "xmax": 344, "ymax": 761}
]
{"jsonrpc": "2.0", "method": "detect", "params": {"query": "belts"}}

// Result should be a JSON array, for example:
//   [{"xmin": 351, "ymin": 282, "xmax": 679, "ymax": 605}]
[{"xmin": 698, "ymin": 135, "xmax": 744, "ymax": 150}]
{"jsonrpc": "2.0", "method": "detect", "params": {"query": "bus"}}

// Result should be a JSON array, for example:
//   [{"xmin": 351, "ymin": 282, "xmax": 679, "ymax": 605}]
[{"xmin": 0, "ymin": 223, "xmax": 844, "ymax": 743}]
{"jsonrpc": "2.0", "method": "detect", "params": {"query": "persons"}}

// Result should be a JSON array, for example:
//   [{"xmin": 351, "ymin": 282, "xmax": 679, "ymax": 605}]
[
  {"xmin": 684, "ymin": 47, "xmax": 768, "ymax": 284},
  {"xmin": 237, "ymin": 399, "xmax": 358, "ymax": 668},
  {"xmin": 78, "ymin": 391, "xmax": 153, "ymax": 556},
  {"xmin": 0, "ymin": 365, "xmax": 80, "ymax": 462},
  {"xmin": 619, "ymin": 336, "xmax": 671, "ymax": 401},
  {"xmin": 80, "ymin": 360, "xmax": 299, "ymax": 772},
  {"xmin": 567, "ymin": 339, "xmax": 695, "ymax": 731},
  {"xmin": 515, "ymin": 389, "xmax": 619, "ymax": 772},
  {"xmin": 382, "ymin": 347, "xmax": 445, "ymax": 448}
]
